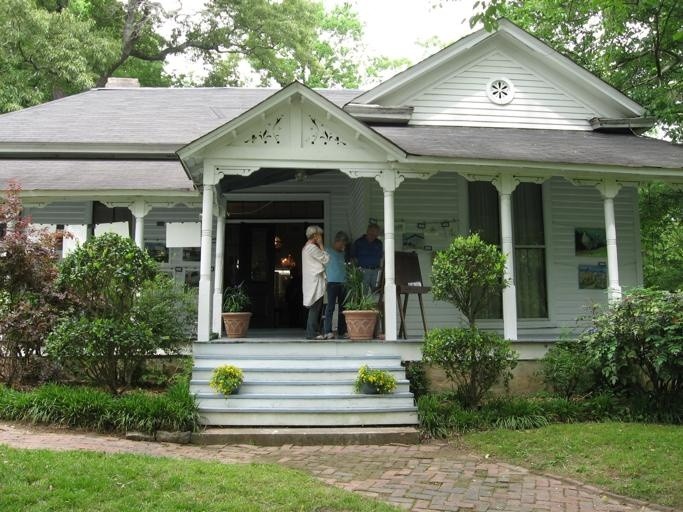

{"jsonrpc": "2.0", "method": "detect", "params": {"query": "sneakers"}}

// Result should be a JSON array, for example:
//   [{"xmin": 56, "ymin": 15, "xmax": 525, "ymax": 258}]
[
  {"xmin": 310, "ymin": 334, "xmax": 327, "ymax": 340},
  {"xmin": 324, "ymin": 332, "xmax": 335, "ymax": 339},
  {"xmin": 337, "ymin": 333, "xmax": 350, "ymax": 339}
]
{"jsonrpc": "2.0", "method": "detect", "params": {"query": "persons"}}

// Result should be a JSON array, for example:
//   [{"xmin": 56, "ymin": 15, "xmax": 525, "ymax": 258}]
[
  {"xmin": 324, "ymin": 231, "xmax": 349, "ymax": 336},
  {"xmin": 301, "ymin": 225, "xmax": 330, "ymax": 339},
  {"xmin": 347, "ymin": 224, "xmax": 383, "ymax": 295}
]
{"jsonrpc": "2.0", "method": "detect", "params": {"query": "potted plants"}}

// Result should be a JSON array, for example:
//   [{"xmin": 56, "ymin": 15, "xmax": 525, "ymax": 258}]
[
  {"xmin": 353, "ymin": 364, "xmax": 397, "ymax": 397},
  {"xmin": 206, "ymin": 366, "xmax": 243, "ymax": 397},
  {"xmin": 220, "ymin": 282, "xmax": 252, "ymax": 339},
  {"xmin": 341, "ymin": 261, "xmax": 381, "ymax": 341}
]
